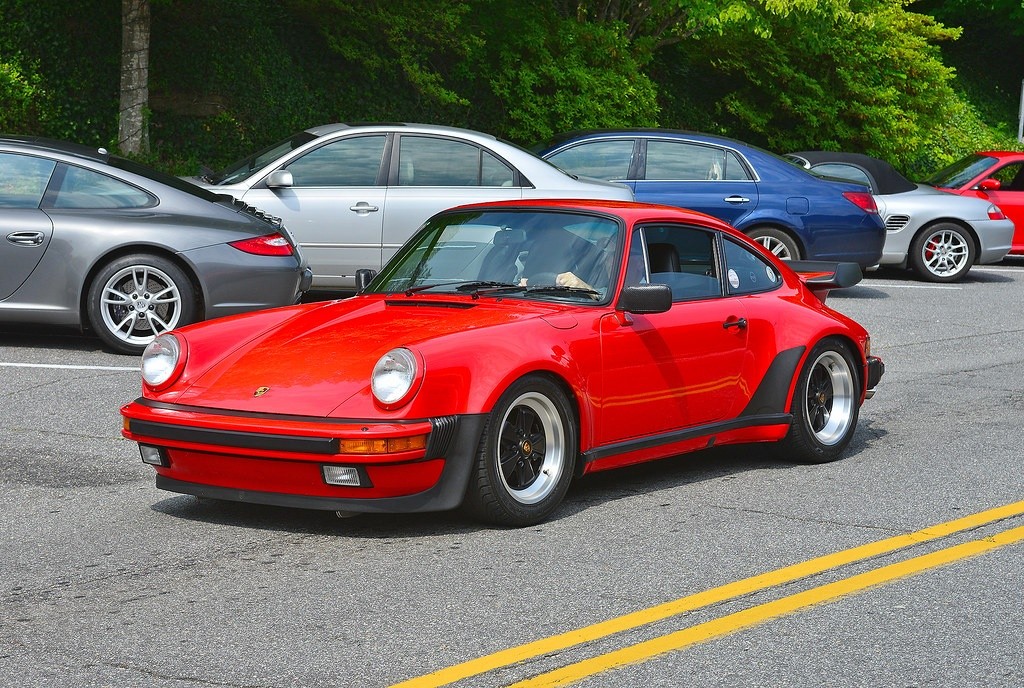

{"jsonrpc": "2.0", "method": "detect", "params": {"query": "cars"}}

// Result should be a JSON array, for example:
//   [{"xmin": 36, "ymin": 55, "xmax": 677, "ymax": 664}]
[
  {"xmin": 0, "ymin": 135, "xmax": 313, "ymax": 357},
  {"xmin": 176, "ymin": 119, "xmax": 637, "ymax": 293},
  {"xmin": 525, "ymin": 124, "xmax": 886, "ymax": 270},
  {"xmin": 916, "ymin": 150, "xmax": 1024, "ymax": 268},
  {"xmin": 788, "ymin": 150, "xmax": 1015, "ymax": 283}
]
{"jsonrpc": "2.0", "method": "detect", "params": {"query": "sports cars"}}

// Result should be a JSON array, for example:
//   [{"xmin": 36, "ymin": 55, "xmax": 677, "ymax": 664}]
[{"xmin": 118, "ymin": 196, "xmax": 887, "ymax": 531}]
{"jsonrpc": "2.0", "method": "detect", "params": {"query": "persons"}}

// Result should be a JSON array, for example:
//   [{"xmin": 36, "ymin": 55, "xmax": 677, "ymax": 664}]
[{"xmin": 518, "ymin": 228, "xmax": 653, "ymax": 303}]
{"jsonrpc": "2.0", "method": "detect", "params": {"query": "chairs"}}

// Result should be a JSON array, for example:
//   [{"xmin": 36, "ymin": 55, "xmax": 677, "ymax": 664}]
[{"xmin": 649, "ymin": 241, "xmax": 681, "ymax": 291}]
